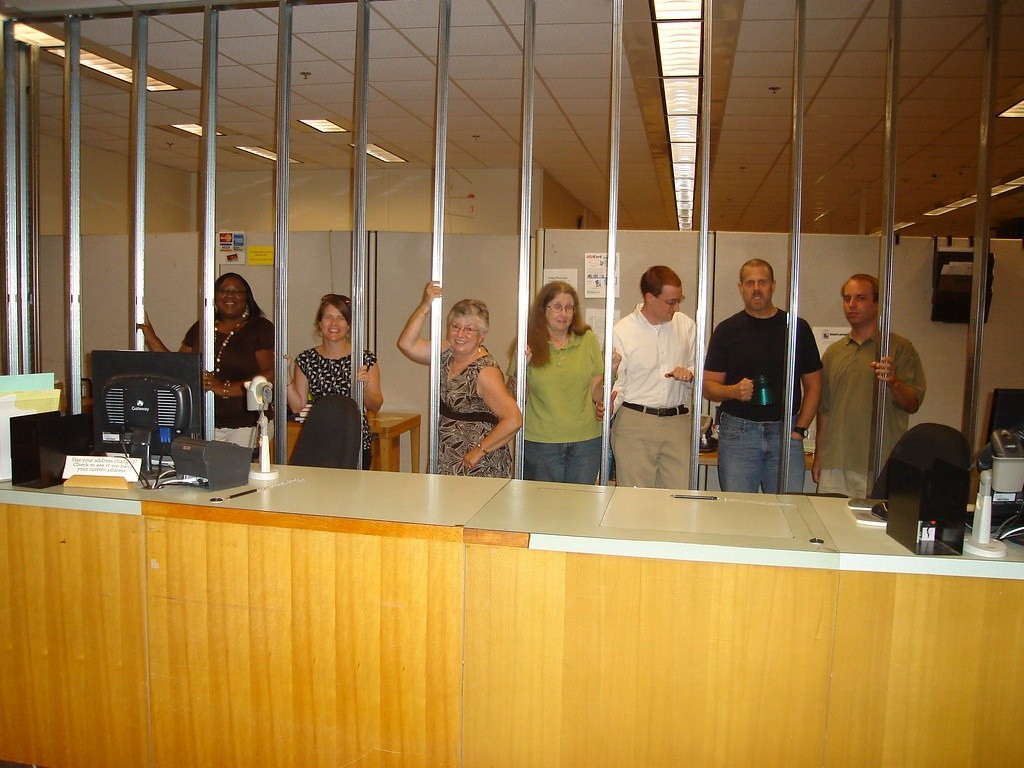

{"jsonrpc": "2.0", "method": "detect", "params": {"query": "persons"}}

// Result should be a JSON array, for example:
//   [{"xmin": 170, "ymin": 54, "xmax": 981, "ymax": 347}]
[
  {"xmin": 273, "ymin": 293, "xmax": 384, "ymax": 471},
  {"xmin": 397, "ymin": 280, "xmax": 523, "ymax": 479},
  {"xmin": 596, "ymin": 266, "xmax": 707, "ymax": 490},
  {"xmin": 702, "ymin": 258, "xmax": 824, "ymax": 493},
  {"xmin": 136, "ymin": 271, "xmax": 275, "ymax": 450},
  {"xmin": 811, "ymin": 274, "xmax": 927, "ymax": 498},
  {"xmin": 506, "ymin": 281, "xmax": 622, "ymax": 486}
]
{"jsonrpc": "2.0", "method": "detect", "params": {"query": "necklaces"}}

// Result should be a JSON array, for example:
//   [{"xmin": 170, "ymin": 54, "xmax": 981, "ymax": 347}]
[
  {"xmin": 214, "ymin": 307, "xmax": 251, "ymax": 375},
  {"xmin": 451, "ymin": 348, "xmax": 481, "ymax": 377}
]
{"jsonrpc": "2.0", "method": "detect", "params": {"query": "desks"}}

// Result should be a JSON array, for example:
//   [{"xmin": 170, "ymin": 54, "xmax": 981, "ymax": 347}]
[{"xmin": 0, "ymin": 396, "xmax": 1024, "ymax": 768}]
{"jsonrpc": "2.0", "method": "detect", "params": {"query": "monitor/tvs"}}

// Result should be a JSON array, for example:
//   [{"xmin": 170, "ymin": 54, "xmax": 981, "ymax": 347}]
[
  {"xmin": 984, "ymin": 388, "xmax": 1024, "ymax": 444},
  {"xmin": 91, "ymin": 350, "xmax": 205, "ymax": 481}
]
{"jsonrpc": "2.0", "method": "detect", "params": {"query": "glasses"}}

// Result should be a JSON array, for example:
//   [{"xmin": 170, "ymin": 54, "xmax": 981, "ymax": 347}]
[
  {"xmin": 320, "ymin": 294, "xmax": 352, "ymax": 310},
  {"xmin": 545, "ymin": 304, "xmax": 575, "ymax": 313},
  {"xmin": 448, "ymin": 323, "xmax": 479, "ymax": 334},
  {"xmin": 654, "ymin": 293, "xmax": 685, "ymax": 305}
]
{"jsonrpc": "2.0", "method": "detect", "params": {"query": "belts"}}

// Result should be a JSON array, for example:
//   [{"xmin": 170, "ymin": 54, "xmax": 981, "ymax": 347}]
[{"xmin": 623, "ymin": 401, "xmax": 689, "ymax": 417}]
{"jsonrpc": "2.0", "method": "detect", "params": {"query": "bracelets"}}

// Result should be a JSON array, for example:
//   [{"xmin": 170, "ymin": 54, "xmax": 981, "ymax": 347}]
[
  {"xmin": 420, "ymin": 302, "xmax": 430, "ymax": 315},
  {"xmin": 222, "ymin": 380, "xmax": 231, "ymax": 399},
  {"xmin": 287, "ymin": 385, "xmax": 296, "ymax": 391},
  {"xmin": 477, "ymin": 442, "xmax": 489, "ymax": 455},
  {"xmin": 611, "ymin": 372, "xmax": 618, "ymax": 383},
  {"xmin": 287, "ymin": 379, "xmax": 293, "ymax": 388}
]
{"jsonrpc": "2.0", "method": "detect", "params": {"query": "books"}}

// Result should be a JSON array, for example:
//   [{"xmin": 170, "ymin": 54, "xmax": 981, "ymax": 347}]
[{"xmin": 295, "ymin": 390, "xmax": 314, "ymax": 424}]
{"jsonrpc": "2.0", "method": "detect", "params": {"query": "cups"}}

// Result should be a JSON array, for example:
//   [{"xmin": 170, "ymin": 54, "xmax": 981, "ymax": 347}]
[{"xmin": 746, "ymin": 374, "xmax": 774, "ymax": 405}]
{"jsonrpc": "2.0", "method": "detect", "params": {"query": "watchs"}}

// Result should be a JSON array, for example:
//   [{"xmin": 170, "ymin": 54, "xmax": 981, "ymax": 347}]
[{"xmin": 794, "ymin": 426, "xmax": 809, "ymax": 438}]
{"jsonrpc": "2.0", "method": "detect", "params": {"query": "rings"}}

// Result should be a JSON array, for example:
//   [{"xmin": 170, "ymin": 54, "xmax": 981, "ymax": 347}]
[
  {"xmin": 205, "ymin": 372, "xmax": 208, "ymax": 376},
  {"xmin": 683, "ymin": 375, "xmax": 687, "ymax": 378},
  {"xmin": 207, "ymin": 381, "xmax": 210, "ymax": 385}
]
